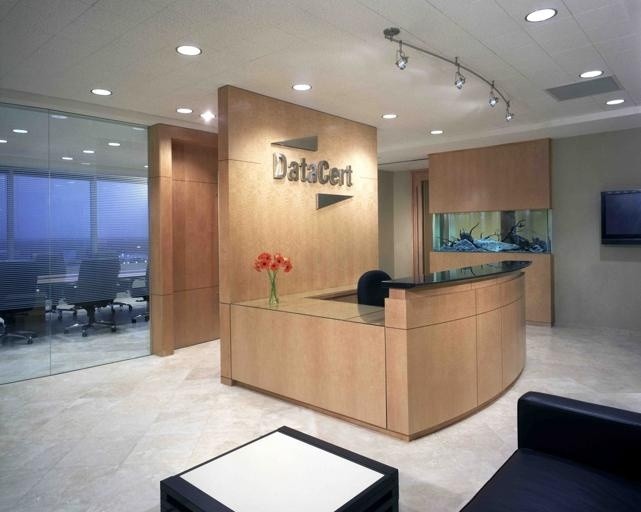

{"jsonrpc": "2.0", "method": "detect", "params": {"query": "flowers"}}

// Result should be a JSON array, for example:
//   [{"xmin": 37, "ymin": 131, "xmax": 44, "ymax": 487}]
[{"xmin": 252, "ymin": 251, "xmax": 293, "ymax": 306}]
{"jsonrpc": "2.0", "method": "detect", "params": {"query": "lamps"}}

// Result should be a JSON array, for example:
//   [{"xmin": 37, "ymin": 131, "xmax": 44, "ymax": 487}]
[
  {"xmin": 487, "ymin": 84, "xmax": 499, "ymax": 108},
  {"xmin": 454, "ymin": 64, "xmax": 466, "ymax": 90},
  {"xmin": 397, "ymin": 40, "xmax": 410, "ymax": 70}
]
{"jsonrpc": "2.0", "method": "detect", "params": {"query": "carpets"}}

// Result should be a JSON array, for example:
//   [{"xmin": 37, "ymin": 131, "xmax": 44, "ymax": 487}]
[{"xmin": 1, "ymin": 299, "xmax": 150, "ymax": 383}]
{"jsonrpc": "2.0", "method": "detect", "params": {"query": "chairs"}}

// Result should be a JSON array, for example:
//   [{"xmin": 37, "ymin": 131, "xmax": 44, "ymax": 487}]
[
  {"xmin": 357, "ymin": 271, "xmax": 392, "ymax": 306},
  {"xmin": 0, "ymin": 251, "xmax": 149, "ymax": 344},
  {"xmin": 457, "ymin": 391, "xmax": 640, "ymax": 511}
]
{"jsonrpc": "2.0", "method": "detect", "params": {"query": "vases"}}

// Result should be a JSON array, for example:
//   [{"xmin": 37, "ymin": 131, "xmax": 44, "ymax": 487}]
[{"xmin": 268, "ymin": 278, "xmax": 279, "ymax": 304}]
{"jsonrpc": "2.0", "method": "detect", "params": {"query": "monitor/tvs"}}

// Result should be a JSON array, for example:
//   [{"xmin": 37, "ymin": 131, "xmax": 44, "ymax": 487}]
[{"xmin": 600, "ymin": 189, "xmax": 641, "ymax": 245}]
